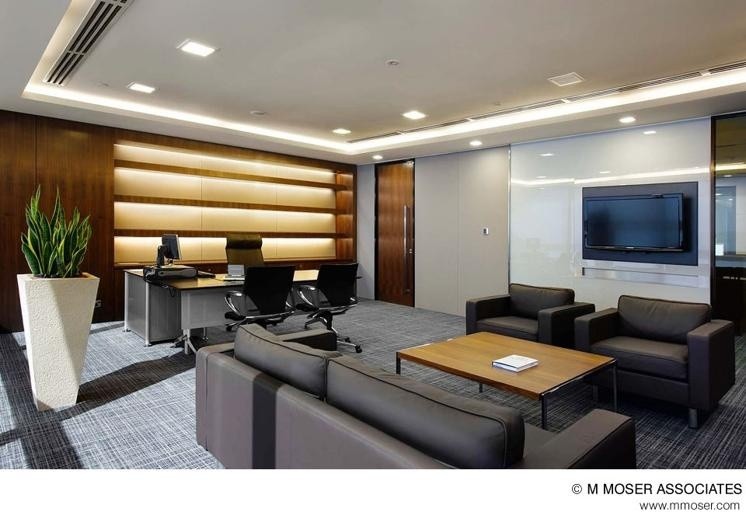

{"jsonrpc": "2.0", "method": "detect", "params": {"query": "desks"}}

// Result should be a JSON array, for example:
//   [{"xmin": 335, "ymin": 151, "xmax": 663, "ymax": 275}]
[{"xmin": 124, "ymin": 269, "xmax": 319, "ymax": 356}]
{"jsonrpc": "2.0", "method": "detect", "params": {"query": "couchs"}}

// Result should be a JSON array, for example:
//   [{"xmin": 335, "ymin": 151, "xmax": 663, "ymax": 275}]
[
  {"xmin": 466, "ymin": 283, "xmax": 595, "ymax": 350},
  {"xmin": 574, "ymin": 295, "xmax": 736, "ymax": 429},
  {"xmin": 195, "ymin": 323, "xmax": 637, "ymax": 469}
]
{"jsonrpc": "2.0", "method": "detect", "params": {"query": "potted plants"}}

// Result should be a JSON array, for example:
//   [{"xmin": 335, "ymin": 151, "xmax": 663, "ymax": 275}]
[{"xmin": 17, "ymin": 184, "xmax": 101, "ymax": 413}]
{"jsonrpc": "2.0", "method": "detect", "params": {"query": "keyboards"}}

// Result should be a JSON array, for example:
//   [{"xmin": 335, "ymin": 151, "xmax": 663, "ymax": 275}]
[{"xmin": 198, "ymin": 271, "xmax": 216, "ymax": 278}]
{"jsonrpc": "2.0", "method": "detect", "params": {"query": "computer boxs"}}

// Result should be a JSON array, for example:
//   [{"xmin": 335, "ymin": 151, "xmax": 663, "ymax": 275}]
[{"xmin": 143, "ymin": 264, "xmax": 198, "ymax": 280}]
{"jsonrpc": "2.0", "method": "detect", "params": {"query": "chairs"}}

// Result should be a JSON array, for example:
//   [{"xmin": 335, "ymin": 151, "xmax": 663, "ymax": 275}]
[
  {"xmin": 225, "ymin": 233, "xmax": 265, "ymax": 273},
  {"xmin": 224, "ymin": 262, "xmax": 295, "ymax": 332},
  {"xmin": 289, "ymin": 263, "xmax": 362, "ymax": 353}
]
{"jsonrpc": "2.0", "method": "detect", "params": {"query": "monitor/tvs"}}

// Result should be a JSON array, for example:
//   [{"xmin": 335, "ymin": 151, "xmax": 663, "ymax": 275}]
[
  {"xmin": 583, "ymin": 192, "xmax": 684, "ymax": 252},
  {"xmin": 157, "ymin": 234, "xmax": 182, "ymax": 265}
]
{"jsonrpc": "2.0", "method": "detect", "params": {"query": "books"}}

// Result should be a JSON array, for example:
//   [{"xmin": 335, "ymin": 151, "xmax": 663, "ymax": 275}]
[{"xmin": 492, "ymin": 355, "xmax": 539, "ymax": 372}]
